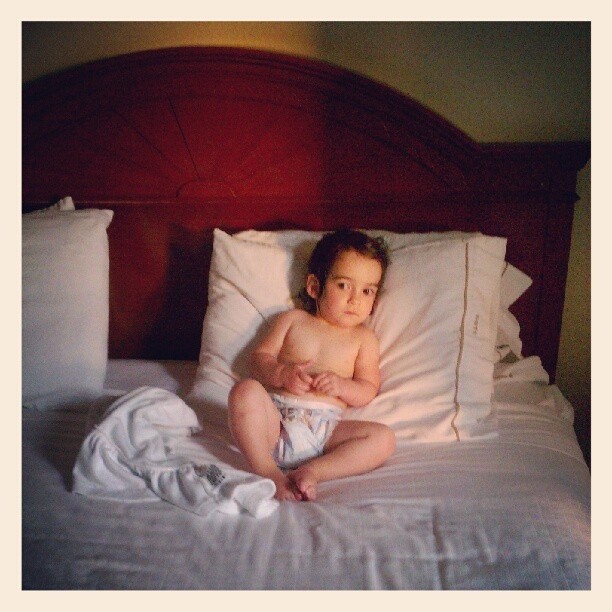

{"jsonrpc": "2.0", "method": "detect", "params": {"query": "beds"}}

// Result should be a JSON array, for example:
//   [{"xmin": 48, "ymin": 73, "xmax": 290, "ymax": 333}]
[{"xmin": 23, "ymin": 47, "xmax": 592, "ymax": 589}]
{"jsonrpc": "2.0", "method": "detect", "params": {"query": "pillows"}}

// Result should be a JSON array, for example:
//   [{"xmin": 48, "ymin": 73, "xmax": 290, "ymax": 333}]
[
  {"xmin": 44, "ymin": 196, "xmax": 76, "ymax": 210},
  {"xmin": 23, "ymin": 208, "xmax": 115, "ymax": 410},
  {"xmin": 232, "ymin": 227, "xmax": 488, "ymax": 255},
  {"xmin": 185, "ymin": 227, "xmax": 508, "ymax": 445}
]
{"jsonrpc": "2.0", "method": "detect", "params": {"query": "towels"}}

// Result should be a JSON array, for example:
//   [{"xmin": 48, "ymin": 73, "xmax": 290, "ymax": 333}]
[{"xmin": 71, "ymin": 384, "xmax": 278, "ymax": 521}]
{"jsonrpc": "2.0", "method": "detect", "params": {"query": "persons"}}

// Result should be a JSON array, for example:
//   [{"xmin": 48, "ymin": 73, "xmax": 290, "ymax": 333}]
[{"xmin": 227, "ymin": 227, "xmax": 398, "ymax": 502}]
